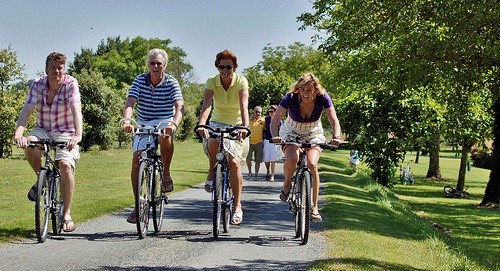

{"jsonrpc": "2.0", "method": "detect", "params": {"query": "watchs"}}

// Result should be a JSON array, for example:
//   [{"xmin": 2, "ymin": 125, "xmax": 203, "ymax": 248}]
[{"xmin": 334, "ymin": 135, "xmax": 340, "ymax": 139}]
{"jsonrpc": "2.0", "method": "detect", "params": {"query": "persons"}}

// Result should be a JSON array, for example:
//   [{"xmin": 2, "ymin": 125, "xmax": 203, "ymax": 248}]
[
  {"xmin": 13, "ymin": 52, "xmax": 83, "ymax": 232},
  {"xmin": 195, "ymin": 50, "xmax": 249, "ymax": 225},
  {"xmin": 122, "ymin": 48, "xmax": 184, "ymax": 224},
  {"xmin": 270, "ymin": 73, "xmax": 341, "ymax": 223},
  {"xmin": 350, "ymin": 148, "xmax": 361, "ymax": 170},
  {"xmin": 262, "ymin": 105, "xmax": 283, "ymax": 182},
  {"xmin": 245, "ymin": 106, "xmax": 266, "ymax": 181}
]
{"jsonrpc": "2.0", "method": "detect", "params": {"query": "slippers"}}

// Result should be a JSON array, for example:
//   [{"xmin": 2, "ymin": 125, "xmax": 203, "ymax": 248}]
[
  {"xmin": 232, "ymin": 209, "xmax": 243, "ymax": 224},
  {"xmin": 28, "ymin": 183, "xmax": 46, "ymax": 201},
  {"xmin": 162, "ymin": 179, "xmax": 174, "ymax": 193},
  {"xmin": 205, "ymin": 179, "xmax": 215, "ymax": 192},
  {"xmin": 62, "ymin": 217, "xmax": 76, "ymax": 232},
  {"xmin": 311, "ymin": 210, "xmax": 322, "ymax": 219},
  {"xmin": 127, "ymin": 212, "xmax": 138, "ymax": 223},
  {"xmin": 280, "ymin": 184, "xmax": 293, "ymax": 202}
]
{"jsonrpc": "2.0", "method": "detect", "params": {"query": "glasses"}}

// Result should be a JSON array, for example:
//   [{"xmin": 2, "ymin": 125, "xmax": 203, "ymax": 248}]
[
  {"xmin": 217, "ymin": 64, "xmax": 236, "ymax": 70},
  {"xmin": 254, "ymin": 111, "xmax": 262, "ymax": 114},
  {"xmin": 149, "ymin": 61, "xmax": 165, "ymax": 65},
  {"xmin": 268, "ymin": 111, "xmax": 275, "ymax": 113}
]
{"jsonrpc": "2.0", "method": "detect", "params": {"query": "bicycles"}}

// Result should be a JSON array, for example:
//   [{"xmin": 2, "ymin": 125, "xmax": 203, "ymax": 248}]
[
  {"xmin": 193, "ymin": 125, "xmax": 253, "ymax": 240},
  {"xmin": 118, "ymin": 117, "xmax": 179, "ymax": 239},
  {"xmin": 444, "ymin": 186, "xmax": 470, "ymax": 199},
  {"xmin": 274, "ymin": 135, "xmax": 339, "ymax": 245},
  {"xmin": 15, "ymin": 138, "xmax": 69, "ymax": 244},
  {"xmin": 400, "ymin": 160, "xmax": 415, "ymax": 185}
]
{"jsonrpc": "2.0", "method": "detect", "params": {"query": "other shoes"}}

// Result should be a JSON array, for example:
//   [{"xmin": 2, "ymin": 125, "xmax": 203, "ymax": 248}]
[
  {"xmin": 246, "ymin": 173, "xmax": 253, "ymax": 179},
  {"xmin": 266, "ymin": 176, "xmax": 270, "ymax": 182},
  {"xmin": 270, "ymin": 176, "xmax": 274, "ymax": 181},
  {"xmin": 254, "ymin": 177, "xmax": 259, "ymax": 181}
]
